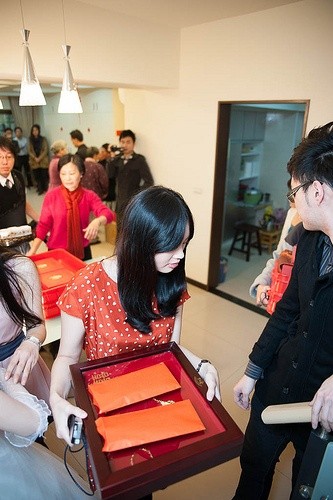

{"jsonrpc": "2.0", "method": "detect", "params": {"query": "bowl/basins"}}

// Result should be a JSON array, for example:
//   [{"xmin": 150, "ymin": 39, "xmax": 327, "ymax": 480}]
[{"xmin": 260, "ymin": 192, "xmax": 270, "ymax": 202}]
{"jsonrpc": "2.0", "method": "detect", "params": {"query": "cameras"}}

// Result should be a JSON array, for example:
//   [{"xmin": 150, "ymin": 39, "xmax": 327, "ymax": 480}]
[{"xmin": 69, "ymin": 414, "xmax": 83, "ymax": 445}]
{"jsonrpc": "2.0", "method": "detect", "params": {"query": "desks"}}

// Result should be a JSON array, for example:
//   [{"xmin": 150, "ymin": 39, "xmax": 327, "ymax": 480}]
[{"xmin": 227, "ymin": 200, "xmax": 275, "ymax": 227}]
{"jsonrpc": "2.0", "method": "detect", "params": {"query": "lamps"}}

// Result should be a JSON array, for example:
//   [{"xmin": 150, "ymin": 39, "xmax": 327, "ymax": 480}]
[
  {"xmin": 18, "ymin": 0, "xmax": 46, "ymax": 106},
  {"xmin": 57, "ymin": 0, "xmax": 83, "ymax": 114}
]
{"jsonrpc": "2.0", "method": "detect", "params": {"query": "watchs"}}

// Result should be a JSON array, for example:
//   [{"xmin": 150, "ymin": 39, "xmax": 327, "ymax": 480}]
[{"xmin": 25, "ymin": 336, "xmax": 43, "ymax": 347}]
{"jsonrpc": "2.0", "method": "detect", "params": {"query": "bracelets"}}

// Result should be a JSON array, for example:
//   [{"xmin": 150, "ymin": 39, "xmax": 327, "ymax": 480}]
[{"xmin": 196, "ymin": 360, "xmax": 213, "ymax": 372}]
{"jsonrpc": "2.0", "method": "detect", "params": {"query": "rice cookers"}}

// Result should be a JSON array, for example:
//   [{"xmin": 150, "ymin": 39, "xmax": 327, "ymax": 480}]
[{"xmin": 244, "ymin": 187, "xmax": 261, "ymax": 205}]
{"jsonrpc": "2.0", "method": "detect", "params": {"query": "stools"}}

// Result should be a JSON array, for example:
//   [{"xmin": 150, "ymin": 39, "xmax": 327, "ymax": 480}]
[
  {"xmin": 259, "ymin": 227, "xmax": 280, "ymax": 252},
  {"xmin": 229, "ymin": 222, "xmax": 263, "ymax": 262}
]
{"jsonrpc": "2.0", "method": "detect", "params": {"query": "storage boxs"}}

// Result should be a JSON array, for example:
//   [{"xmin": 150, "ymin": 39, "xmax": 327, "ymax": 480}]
[
  {"xmin": 73, "ymin": 342, "xmax": 245, "ymax": 498},
  {"xmin": 25, "ymin": 248, "xmax": 89, "ymax": 319}
]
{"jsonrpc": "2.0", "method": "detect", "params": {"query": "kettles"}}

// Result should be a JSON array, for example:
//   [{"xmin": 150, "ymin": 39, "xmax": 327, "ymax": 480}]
[{"xmin": 259, "ymin": 214, "xmax": 276, "ymax": 233}]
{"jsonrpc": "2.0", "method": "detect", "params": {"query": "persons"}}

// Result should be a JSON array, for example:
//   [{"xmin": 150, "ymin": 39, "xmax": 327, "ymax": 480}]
[
  {"xmin": 48, "ymin": 186, "xmax": 221, "ymax": 450},
  {"xmin": 249, "ymin": 177, "xmax": 300, "ymax": 308},
  {"xmin": 234, "ymin": 120, "xmax": 333, "ymax": 500},
  {"xmin": 0, "ymin": 124, "xmax": 156, "ymax": 242},
  {"xmin": 0, "ymin": 366, "xmax": 95, "ymax": 500},
  {"xmin": 0, "ymin": 242, "xmax": 57, "ymax": 451},
  {"xmin": 26, "ymin": 154, "xmax": 115, "ymax": 261}
]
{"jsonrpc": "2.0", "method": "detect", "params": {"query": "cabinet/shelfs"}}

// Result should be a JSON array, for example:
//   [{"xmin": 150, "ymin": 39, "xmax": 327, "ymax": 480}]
[
  {"xmin": 226, "ymin": 137, "xmax": 262, "ymax": 203},
  {"xmin": 230, "ymin": 111, "xmax": 267, "ymax": 141}
]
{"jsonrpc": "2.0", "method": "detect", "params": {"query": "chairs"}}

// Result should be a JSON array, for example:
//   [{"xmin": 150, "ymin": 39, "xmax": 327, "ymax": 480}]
[{"xmin": 91, "ymin": 220, "xmax": 118, "ymax": 259}]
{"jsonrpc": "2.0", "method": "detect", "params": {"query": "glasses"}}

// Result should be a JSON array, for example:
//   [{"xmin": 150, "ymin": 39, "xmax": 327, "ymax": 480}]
[
  {"xmin": 287, "ymin": 180, "xmax": 323, "ymax": 203},
  {"xmin": 0, "ymin": 155, "xmax": 14, "ymax": 160}
]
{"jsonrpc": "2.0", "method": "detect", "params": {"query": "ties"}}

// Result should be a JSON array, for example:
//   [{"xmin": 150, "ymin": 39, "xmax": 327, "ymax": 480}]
[{"xmin": 5, "ymin": 179, "xmax": 10, "ymax": 189}]
